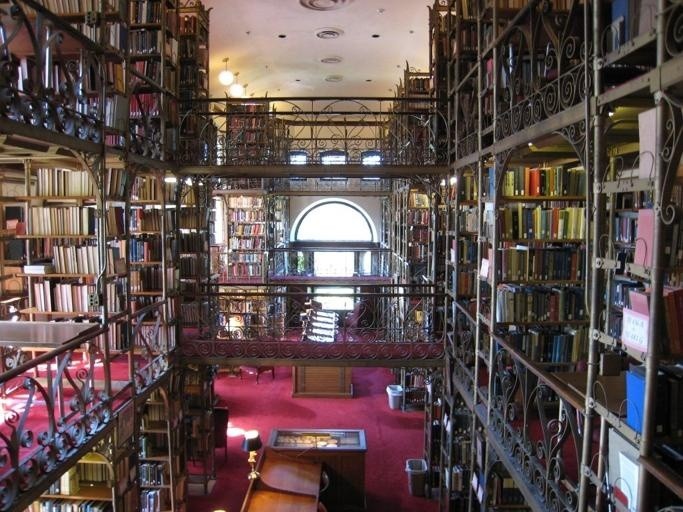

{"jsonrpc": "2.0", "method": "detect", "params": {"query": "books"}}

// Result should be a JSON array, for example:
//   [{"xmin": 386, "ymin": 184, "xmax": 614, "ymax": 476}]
[
  {"xmin": 450, "ymin": 209, "xmax": 478, "ymax": 232},
  {"xmin": 181, "ymin": 256, "xmax": 209, "ymax": 276},
  {"xmin": 179, "ymin": 231, "xmax": 209, "ymax": 252},
  {"xmin": 463, "ymin": 1, "xmax": 478, "ymax": 18},
  {"xmin": 603, "ymin": 279, "xmax": 643, "ymax": 308},
  {"xmin": 634, "ymin": 208, "xmax": 683, "ymax": 269},
  {"xmin": 182, "ymin": 64, "xmax": 199, "ymax": 89},
  {"xmin": 487, "ymin": 473, "xmax": 525, "ymax": 505},
  {"xmin": 180, "ymin": 36, "xmax": 193, "ymax": 59},
  {"xmin": 36, "ymin": 167, "xmax": 126, "ymax": 196},
  {"xmin": 34, "ymin": 279, "xmax": 127, "ymax": 313},
  {"xmin": 52, "ymin": 316, "xmax": 127, "ymax": 350},
  {"xmin": 181, "ymin": 15, "xmax": 196, "ymax": 34},
  {"xmin": 4, "ymin": 239, "xmax": 24, "ymax": 260},
  {"xmin": 229, "ymin": 224, "xmax": 265, "ymax": 235},
  {"xmin": 409, "ymin": 77, "xmax": 430, "ymax": 91},
  {"xmin": 181, "ymin": 184, "xmax": 195, "ymax": 204},
  {"xmin": 460, "ymin": 23, "xmax": 478, "ymax": 51},
  {"xmin": 128, "ymin": 29, "xmax": 171, "ymax": 55},
  {"xmin": 182, "ymin": 302, "xmax": 209, "ymax": 328},
  {"xmin": 143, "ymin": 403, "xmax": 168, "ymax": 431},
  {"xmin": 129, "ymin": 93, "xmax": 160, "ymax": 116},
  {"xmin": 72, "ymin": 21, "xmax": 127, "ymax": 51},
  {"xmin": 128, "ymin": 264, "xmax": 179, "ymax": 293},
  {"xmin": 128, "ymin": 60, "xmax": 172, "ymax": 90},
  {"xmin": 451, "ymin": 238, "xmax": 477, "ymax": 264},
  {"xmin": 24, "ymin": 238, "xmax": 127, "ymax": 274},
  {"xmin": 495, "ymin": 326, "xmax": 586, "ymax": 361},
  {"xmin": 42, "ymin": 456, "xmax": 129, "ymax": 495},
  {"xmin": 442, "ymin": 467, "xmax": 469, "ymax": 492},
  {"xmin": 181, "ymin": 280, "xmax": 208, "ymax": 297},
  {"xmin": 137, "ymin": 462, "xmax": 169, "ymax": 486},
  {"xmin": 231, "ymin": 263, "xmax": 262, "ymax": 277},
  {"xmin": 179, "ymin": 207, "xmax": 207, "ymax": 229},
  {"xmin": 230, "ymin": 104, "xmax": 265, "ymax": 113},
  {"xmin": 481, "ymin": 244, "xmax": 586, "ymax": 281},
  {"xmin": 230, "ymin": 251, "xmax": 262, "ymax": 262},
  {"xmin": 76, "ymin": 94, "xmax": 127, "ymax": 145},
  {"xmin": 131, "ymin": 296, "xmax": 179, "ymax": 320},
  {"xmin": 624, "ymin": 363, "xmax": 682, "ymax": 437},
  {"xmin": 6, "ymin": 206, "xmax": 24, "ymax": 231},
  {"xmin": 495, "ymin": 283, "xmax": 585, "ymax": 322},
  {"xmin": 141, "ymin": 490, "xmax": 166, "ymax": 512},
  {"xmin": 228, "ymin": 209, "xmax": 266, "ymax": 222},
  {"xmin": 228, "ymin": 236, "xmax": 265, "ymax": 249},
  {"xmin": 29, "ymin": 205, "xmax": 124, "ymax": 236},
  {"xmin": 22, "ymin": 499, "xmax": 113, "ymax": 512},
  {"xmin": 490, "ymin": 0, "xmax": 573, "ymax": 10},
  {"xmin": 16, "ymin": 58, "xmax": 126, "ymax": 93},
  {"xmin": 129, "ymin": 1, "xmax": 167, "ymax": 24},
  {"xmin": 4, "ymin": 265, "xmax": 24, "ymax": 296},
  {"xmin": 131, "ymin": 176, "xmax": 178, "ymax": 200},
  {"xmin": 231, "ymin": 116, "xmax": 265, "ymax": 128},
  {"xmin": 630, "ymin": 287, "xmax": 683, "ymax": 356},
  {"xmin": 480, "ymin": 205, "xmax": 586, "ymax": 240},
  {"xmin": 485, "ymin": 165, "xmax": 587, "ymax": 196},
  {"xmin": 608, "ymin": 208, "xmax": 638, "ymax": 243},
  {"xmin": 11, "ymin": 0, "xmax": 120, "ymax": 14},
  {"xmin": 128, "ymin": 233, "xmax": 177, "ymax": 263},
  {"xmin": 409, "ymin": 94, "xmax": 430, "ymax": 110},
  {"xmin": 451, "ymin": 270, "xmax": 477, "ymax": 295},
  {"xmin": 132, "ymin": 325, "xmax": 175, "ymax": 348},
  {"xmin": 129, "ymin": 208, "xmax": 176, "ymax": 231},
  {"xmin": 231, "ymin": 131, "xmax": 265, "ymax": 143},
  {"xmin": 137, "ymin": 434, "xmax": 169, "ymax": 459}
]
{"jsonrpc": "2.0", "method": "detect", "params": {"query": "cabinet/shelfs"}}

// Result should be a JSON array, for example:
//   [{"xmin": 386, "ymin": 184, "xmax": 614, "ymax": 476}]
[
  {"xmin": 221, "ymin": 96, "xmax": 290, "ymax": 168},
  {"xmin": 379, "ymin": 176, "xmax": 430, "ymax": 344},
  {"xmin": 430, "ymin": 0, "xmax": 683, "ymax": 511},
  {"xmin": 213, "ymin": 178, "xmax": 288, "ymax": 341},
  {"xmin": 380, "ymin": 66, "xmax": 431, "ymax": 165},
  {"xmin": 1, "ymin": 0, "xmax": 211, "ymax": 512}
]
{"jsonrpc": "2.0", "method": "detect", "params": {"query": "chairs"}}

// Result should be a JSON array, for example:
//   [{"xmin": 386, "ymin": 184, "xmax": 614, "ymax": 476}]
[
  {"xmin": 238, "ymin": 365, "xmax": 274, "ymax": 384},
  {"xmin": 211, "ymin": 405, "xmax": 229, "ymax": 460}
]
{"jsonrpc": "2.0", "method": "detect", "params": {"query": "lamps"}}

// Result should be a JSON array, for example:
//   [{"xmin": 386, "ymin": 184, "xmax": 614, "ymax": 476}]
[{"xmin": 241, "ymin": 429, "xmax": 262, "ymax": 480}]
